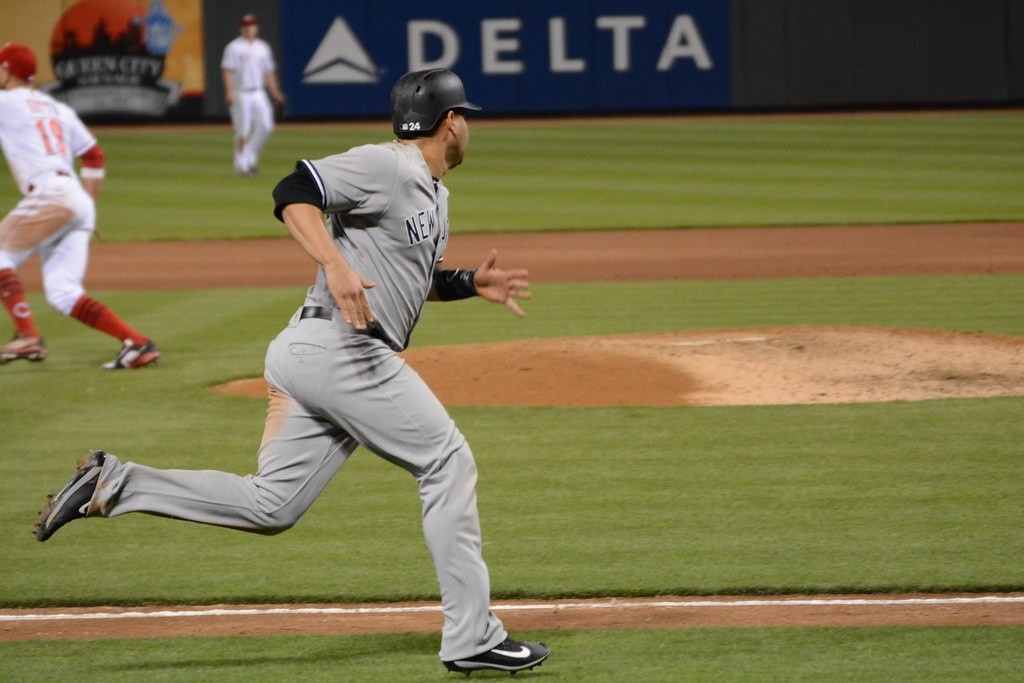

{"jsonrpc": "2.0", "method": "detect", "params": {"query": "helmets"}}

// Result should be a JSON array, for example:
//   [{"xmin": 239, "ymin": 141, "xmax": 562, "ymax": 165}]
[{"xmin": 390, "ymin": 68, "xmax": 484, "ymax": 136}]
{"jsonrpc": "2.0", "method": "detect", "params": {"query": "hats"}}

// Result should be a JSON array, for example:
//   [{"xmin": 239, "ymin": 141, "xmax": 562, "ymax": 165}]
[
  {"xmin": 240, "ymin": 14, "xmax": 257, "ymax": 26},
  {"xmin": 0, "ymin": 41, "xmax": 38, "ymax": 81}
]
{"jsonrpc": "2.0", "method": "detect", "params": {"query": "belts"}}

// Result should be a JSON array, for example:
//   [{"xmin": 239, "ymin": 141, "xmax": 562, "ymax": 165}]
[
  {"xmin": 28, "ymin": 170, "xmax": 70, "ymax": 191},
  {"xmin": 301, "ymin": 305, "xmax": 388, "ymax": 342}
]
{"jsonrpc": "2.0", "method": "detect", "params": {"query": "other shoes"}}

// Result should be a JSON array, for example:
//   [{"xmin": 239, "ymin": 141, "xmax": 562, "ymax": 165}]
[{"xmin": 235, "ymin": 154, "xmax": 258, "ymax": 173}]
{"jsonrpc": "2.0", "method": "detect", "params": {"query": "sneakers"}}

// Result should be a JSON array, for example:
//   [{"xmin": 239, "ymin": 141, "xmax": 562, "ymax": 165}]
[
  {"xmin": 443, "ymin": 636, "xmax": 550, "ymax": 678},
  {"xmin": 0, "ymin": 335, "xmax": 48, "ymax": 364},
  {"xmin": 31, "ymin": 449, "xmax": 106, "ymax": 542},
  {"xmin": 102, "ymin": 338, "xmax": 161, "ymax": 369}
]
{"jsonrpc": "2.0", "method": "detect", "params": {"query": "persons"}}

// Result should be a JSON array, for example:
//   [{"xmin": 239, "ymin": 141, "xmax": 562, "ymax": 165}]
[
  {"xmin": 219, "ymin": 14, "xmax": 285, "ymax": 178},
  {"xmin": 31, "ymin": 68, "xmax": 552, "ymax": 677},
  {"xmin": 0, "ymin": 41, "xmax": 161, "ymax": 370}
]
